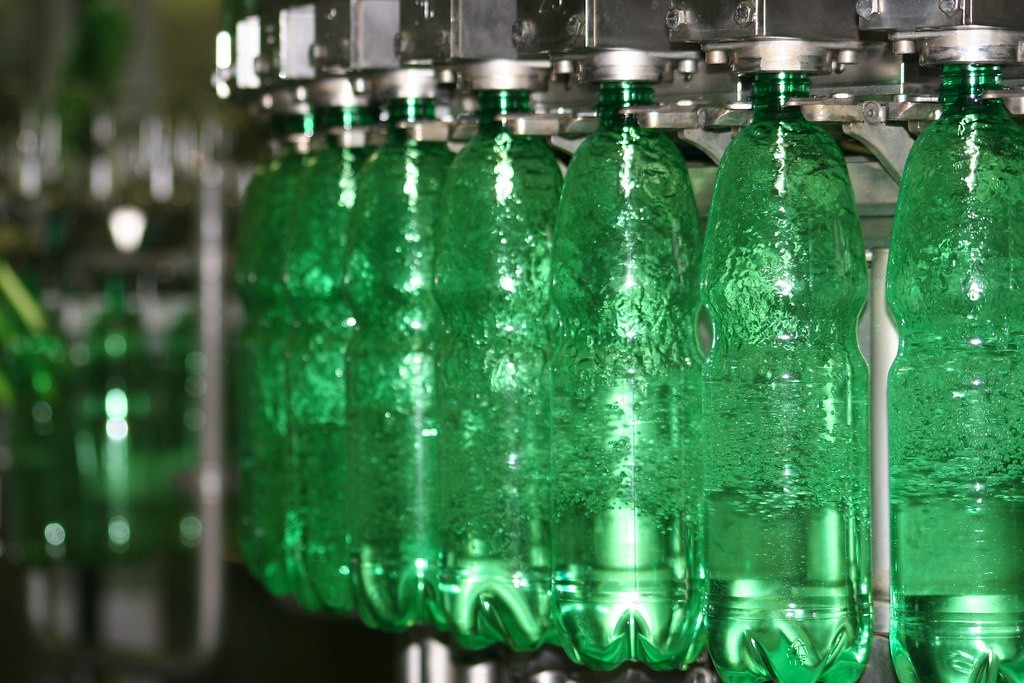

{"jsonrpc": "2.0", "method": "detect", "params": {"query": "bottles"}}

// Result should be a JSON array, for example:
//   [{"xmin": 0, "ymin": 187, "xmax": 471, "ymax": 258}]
[
  {"xmin": 556, "ymin": 47, "xmax": 708, "ymax": 669},
  {"xmin": 700, "ymin": 35, "xmax": 873, "ymax": 683},
  {"xmin": 887, "ymin": 24, "xmax": 1024, "ymax": 683},
  {"xmin": 0, "ymin": 271, "xmax": 197, "ymax": 565},
  {"xmin": 233, "ymin": 80, "xmax": 359, "ymax": 611},
  {"xmin": 340, "ymin": 69, "xmax": 451, "ymax": 632},
  {"xmin": 445, "ymin": 59, "xmax": 565, "ymax": 653}
]
